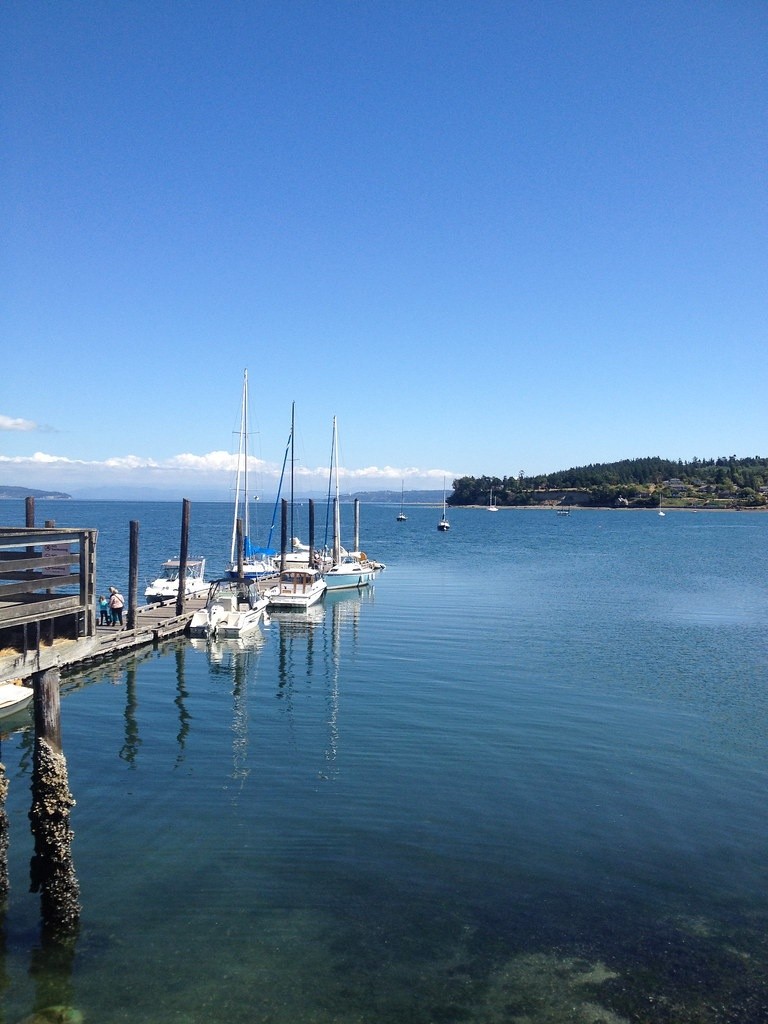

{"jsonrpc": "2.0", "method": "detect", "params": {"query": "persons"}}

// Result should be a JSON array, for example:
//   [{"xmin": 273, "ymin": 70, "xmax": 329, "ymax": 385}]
[
  {"xmin": 108, "ymin": 589, "xmax": 124, "ymax": 626},
  {"xmin": 98, "ymin": 595, "xmax": 109, "ymax": 626},
  {"xmin": 109, "ymin": 587, "xmax": 119, "ymax": 621}
]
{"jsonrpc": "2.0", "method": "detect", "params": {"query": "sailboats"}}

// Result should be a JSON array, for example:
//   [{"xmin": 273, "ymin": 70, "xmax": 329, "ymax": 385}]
[
  {"xmin": 436, "ymin": 475, "xmax": 451, "ymax": 531},
  {"xmin": 396, "ymin": 479, "xmax": 408, "ymax": 522},
  {"xmin": 487, "ymin": 488, "xmax": 499, "ymax": 512},
  {"xmin": 657, "ymin": 493, "xmax": 666, "ymax": 516},
  {"xmin": 189, "ymin": 366, "xmax": 387, "ymax": 640}
]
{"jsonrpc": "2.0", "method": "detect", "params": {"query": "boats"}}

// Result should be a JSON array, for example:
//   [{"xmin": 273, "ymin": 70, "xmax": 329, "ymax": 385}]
[
  {"xmin": 556, "ymin": 509, "xmax": 570, "ymax": 516},
  {"xmin": 144, "ymin": 554, "xmax": 212, "ymax": 605}
]
